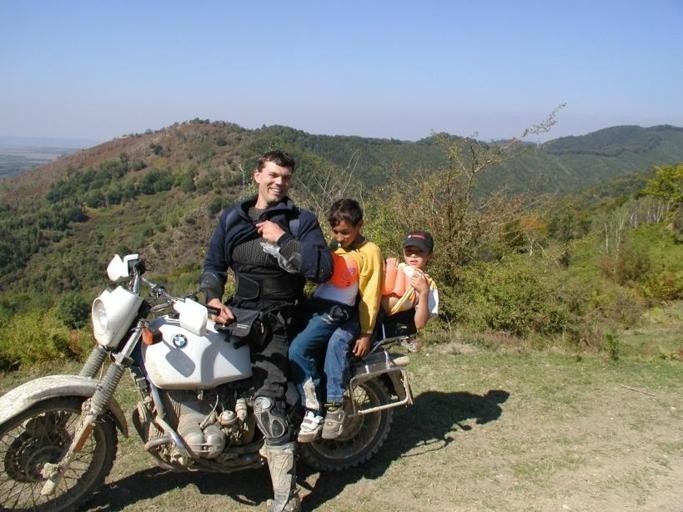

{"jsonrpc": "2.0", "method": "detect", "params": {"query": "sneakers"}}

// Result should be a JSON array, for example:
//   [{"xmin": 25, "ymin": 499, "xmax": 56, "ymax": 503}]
[
  {"xmin": 297, "ymin": 410, "xmax": 323, "ymax": 443},
  {"xmin": 321, "ymin": 408, "xmax": 345, "ymax": 440}
]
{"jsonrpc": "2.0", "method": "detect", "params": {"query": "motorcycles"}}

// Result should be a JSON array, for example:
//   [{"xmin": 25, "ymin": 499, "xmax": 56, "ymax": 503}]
[{"xmin": 0, "ymin": 251, "xmax": 423, "ymax": 512}]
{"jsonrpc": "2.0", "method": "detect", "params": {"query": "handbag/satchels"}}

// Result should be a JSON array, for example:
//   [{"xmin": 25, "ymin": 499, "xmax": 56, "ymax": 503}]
[{"xmin": 215, "ymin": 305, "xmax": 270, "ymax": 349}]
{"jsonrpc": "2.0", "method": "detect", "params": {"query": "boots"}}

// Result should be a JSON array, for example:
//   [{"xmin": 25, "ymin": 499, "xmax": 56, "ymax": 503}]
[{"xmin": 264, "ymin": 441, "xmax": 302, "ymax": 512}]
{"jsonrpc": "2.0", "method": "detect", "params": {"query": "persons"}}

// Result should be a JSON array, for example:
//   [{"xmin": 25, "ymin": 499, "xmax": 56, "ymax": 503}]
[
  {"xmin": 286, "ymin": 199, "xmax": 384, "ymax": 444},
  {"xmin": 322, "ymin": 231, "xmax": 440, "ymax": 439},
  {"xmin": 194, "ymin": 147, "xmax": 333, "ymax": 512}
]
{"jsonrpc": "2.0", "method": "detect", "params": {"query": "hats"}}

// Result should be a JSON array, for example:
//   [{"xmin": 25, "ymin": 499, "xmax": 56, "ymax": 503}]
[{"xmin": 402, "ymin": 232, "xmax": 432, "ymax": 252}]
